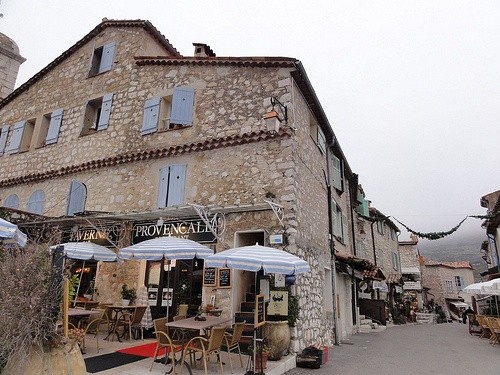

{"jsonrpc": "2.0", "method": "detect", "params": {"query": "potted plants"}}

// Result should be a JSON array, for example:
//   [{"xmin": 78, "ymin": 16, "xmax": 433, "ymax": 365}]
[
  {"xmin": 121, "ymin": 284, "xmax": 136, "ymax": 304},
  {"xmin": 264, "ymin": 290, "xmax": 300, "ymax": 356}
]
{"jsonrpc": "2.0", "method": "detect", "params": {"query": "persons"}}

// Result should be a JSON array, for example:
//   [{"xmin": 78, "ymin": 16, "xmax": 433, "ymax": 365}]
[
  {"xmin": 427, "ymin": 298, "xmax": 447, "ymax": 323},
  {"xmin": 462, "ymin": 306, "xmax": 474, "ymax": 324}
]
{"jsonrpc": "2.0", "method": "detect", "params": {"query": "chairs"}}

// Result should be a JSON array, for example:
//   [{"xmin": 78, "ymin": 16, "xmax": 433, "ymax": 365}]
[
  {"xmin": 71, "ymin": 304, "xmax": 147, "ymax": 353},
  {"xmin": 475, "ymin": 315, "xmax": 500, "ymax": 346},
  {"xmin": 149, "ymin": 315, "xmax": 246, "ymax": 375}
]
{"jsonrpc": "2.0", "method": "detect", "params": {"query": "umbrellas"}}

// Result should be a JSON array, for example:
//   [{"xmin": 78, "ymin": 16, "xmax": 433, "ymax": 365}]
[
  {"xmin": 118, "ymin": 235, "xmax": 215, "ymax": 359},
  {"xmin": 48, "ymin": 242, "xmax": 118, "ymax": 307},
  {"xmin": 204, "ymin": 242, "xmax": 313, "ymax": 297},
  {"xmin": 136, "ymin": 285, "xmax": 154, "ymax": 341},
  {"xmin": 462, "ymin": 277, "xmax": 500, "ymax": 318}
]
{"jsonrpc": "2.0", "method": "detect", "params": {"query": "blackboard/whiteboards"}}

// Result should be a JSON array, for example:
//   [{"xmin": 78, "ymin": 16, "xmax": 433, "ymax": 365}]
[
  {"xmin": 203, "ymin": 267, "xmax": 217, "ymax": 287},
  {"xmin": 218, "ymin": 269, "xmax": 231, "ymax": 288}
]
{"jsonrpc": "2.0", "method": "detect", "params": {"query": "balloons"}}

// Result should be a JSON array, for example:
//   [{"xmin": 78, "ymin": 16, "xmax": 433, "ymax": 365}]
[{"xmin": 0, "ymin": 217, "xmax": 27, "ymax": 249}]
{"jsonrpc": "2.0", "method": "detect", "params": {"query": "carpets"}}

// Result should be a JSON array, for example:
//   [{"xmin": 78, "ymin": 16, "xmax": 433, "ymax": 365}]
[
  {"xmin": 117, "ymin": 342, "xmax": 187, "ymax": 357},
  {"xmin": 85, "ymin": 352, "xmax": 148, "ymax": 373}
]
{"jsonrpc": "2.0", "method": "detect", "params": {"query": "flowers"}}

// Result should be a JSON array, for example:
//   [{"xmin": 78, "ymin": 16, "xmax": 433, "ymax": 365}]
[{"xmin": 248, "ymin": 344, "xmax": 275, "ymax": 358}]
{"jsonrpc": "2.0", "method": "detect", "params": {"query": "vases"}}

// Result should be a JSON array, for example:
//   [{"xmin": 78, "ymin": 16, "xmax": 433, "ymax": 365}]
[{"xmin": 251, "ymin": 352, "xmax": 267, "ymax": 369}]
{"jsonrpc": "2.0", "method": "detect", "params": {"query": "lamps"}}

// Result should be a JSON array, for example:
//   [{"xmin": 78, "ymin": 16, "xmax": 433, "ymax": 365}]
[
  {"xmin": 73, "ymin": 224, "xmax": 79, "ymax": 233},
  {"xmin": 157, "ymin": 217, "xmax": 164, "ymax": 226},
  {"xmin": 263, "ymin": 96, "xmax": 288, "ymax": 138}
]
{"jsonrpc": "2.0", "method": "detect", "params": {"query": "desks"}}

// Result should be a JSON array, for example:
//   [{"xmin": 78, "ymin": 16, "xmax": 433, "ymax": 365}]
[
  {"xmin": 103, "ymin": 305, "xmax": 138, "ymax": 341},
  {"xmin": 68, "ymin": 310, "xmax": 100, "ymax": 354},
  {"xmin": 165, "ymin": 315, "xmax": 232, "ymax": 375},
  {"xmin": 72, "ymin": 300, "xmax": 99, "ymax": 308}
]
